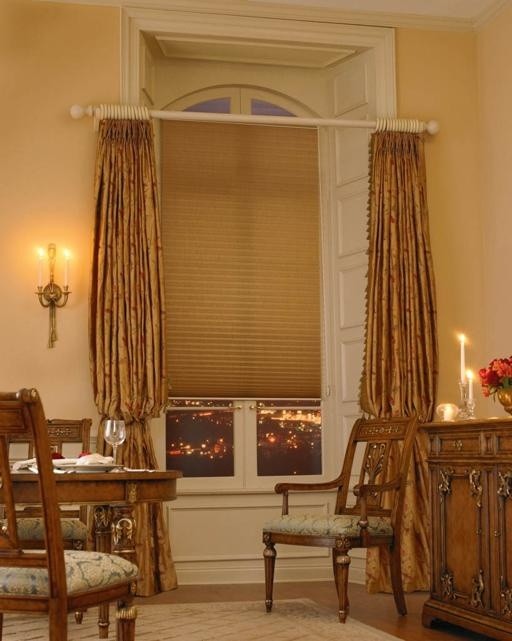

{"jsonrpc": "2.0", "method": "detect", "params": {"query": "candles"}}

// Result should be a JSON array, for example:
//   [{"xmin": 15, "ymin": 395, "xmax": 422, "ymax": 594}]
[
  {"xmin": 460, "ymin": 335, "xmax": 465, "ymax": 384},
  {"xmin": 466, "ymin": 370, "xmax": 474, "ymax": 400}
]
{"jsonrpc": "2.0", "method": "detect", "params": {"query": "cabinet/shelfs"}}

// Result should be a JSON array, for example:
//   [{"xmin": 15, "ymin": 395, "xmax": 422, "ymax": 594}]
[{"xmin": 418, "ymin": 417, "xmax": 512, "ymax": 641}]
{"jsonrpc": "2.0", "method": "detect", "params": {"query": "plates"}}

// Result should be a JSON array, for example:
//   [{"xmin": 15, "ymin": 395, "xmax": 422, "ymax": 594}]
[{"xmin": 55, "ymin": 464, "xmax": 123, "ymax": 473}]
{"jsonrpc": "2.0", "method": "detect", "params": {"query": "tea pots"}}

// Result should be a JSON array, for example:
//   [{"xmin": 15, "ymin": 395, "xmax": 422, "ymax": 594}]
[{"xmin": 436, "ymin": 403, "xmax": 462, "ymax": 422}]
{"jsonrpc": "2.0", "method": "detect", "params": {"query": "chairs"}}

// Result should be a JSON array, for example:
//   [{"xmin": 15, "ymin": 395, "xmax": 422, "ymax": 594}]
[
  {"xmin": 262, "ymin": 417, "xmax": 418, "ymax": 623},
  {"xmin": 0, "ymin": 417, "xmax": 93, "ymax": 625},
  {"xmin": 0, "ymin": 387, "xmax": 144, "ymax": 641}
]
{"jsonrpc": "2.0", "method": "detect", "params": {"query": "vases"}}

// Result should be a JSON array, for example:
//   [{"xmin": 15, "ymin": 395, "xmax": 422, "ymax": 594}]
[{"xmin": 498, "ymin": 388, "xmax": 512, "ymax": 415}]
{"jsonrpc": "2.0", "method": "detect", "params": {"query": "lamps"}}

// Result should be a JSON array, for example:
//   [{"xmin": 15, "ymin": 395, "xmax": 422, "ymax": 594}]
[{"xmin": 35, "ymin": 243, "xmax": 70, "ymax": 348}]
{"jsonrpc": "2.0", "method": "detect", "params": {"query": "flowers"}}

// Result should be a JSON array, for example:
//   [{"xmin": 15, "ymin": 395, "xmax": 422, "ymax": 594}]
[{"xmin": 479, "ymin": 356, "xmax": 512, "ymax": 405}]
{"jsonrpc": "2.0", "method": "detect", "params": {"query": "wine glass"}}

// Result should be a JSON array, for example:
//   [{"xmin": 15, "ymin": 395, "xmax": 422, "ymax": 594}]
[{"xmin": 103, "ymin": 420, "xmax": 126, "ymax": 463}]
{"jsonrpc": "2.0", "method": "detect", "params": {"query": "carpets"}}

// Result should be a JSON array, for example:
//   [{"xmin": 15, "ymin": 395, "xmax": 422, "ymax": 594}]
[{"xmin": 0, "ymin": 598, "xmax": 405, "ymax": 641}]
